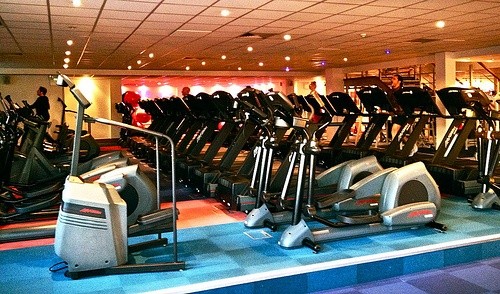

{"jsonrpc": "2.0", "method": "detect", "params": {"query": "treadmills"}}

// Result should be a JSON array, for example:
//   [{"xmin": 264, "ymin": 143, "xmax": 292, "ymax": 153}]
[{"xmin": 287, "ymin": 86, "xmax": 500, "ymax": 197}]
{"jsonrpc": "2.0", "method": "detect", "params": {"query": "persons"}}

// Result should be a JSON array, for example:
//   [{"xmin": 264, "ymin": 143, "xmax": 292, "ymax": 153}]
[
  {"xmin": 181, "ymin": 87, "xmax": 190, "ymax": 98},
  {"xmin": 390, "ymin": 75, "xmax": 418, "ymax": 157},
  {"xmin": 30, "ymin": 86, "xmax": 55, "ymax": 143},
  {"xmin": 309, "ymin": 81, "xmax": 316, "ymax": 94}
]
